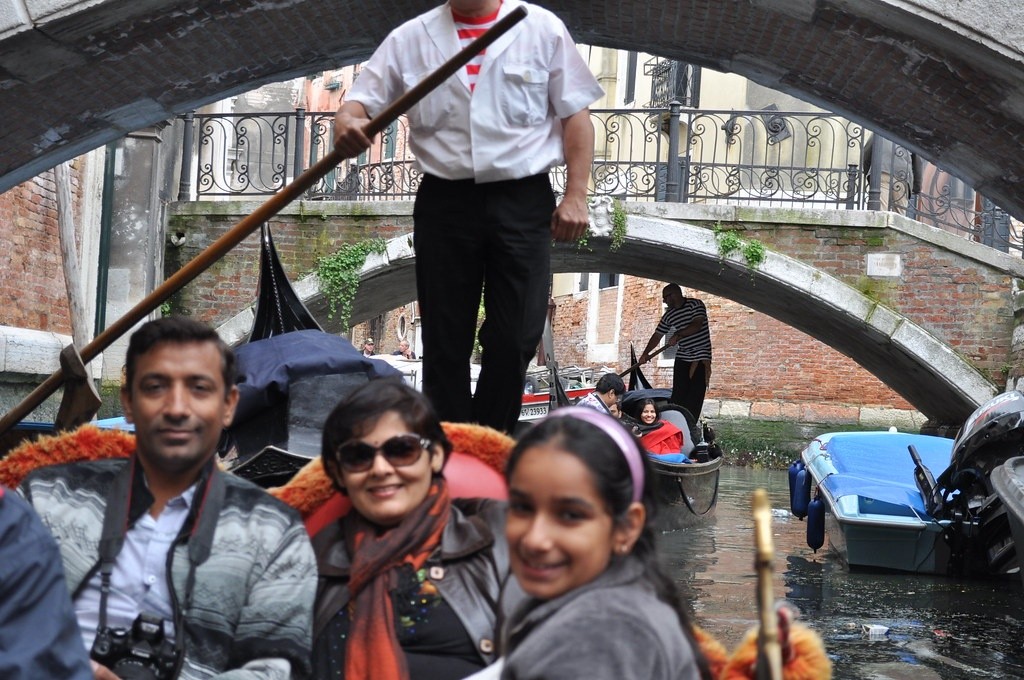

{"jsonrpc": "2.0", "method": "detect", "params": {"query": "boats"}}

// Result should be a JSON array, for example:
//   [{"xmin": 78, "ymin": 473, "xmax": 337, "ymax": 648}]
[
  {"xmin": 517, "ymin": 364, "xmax": 616, "ymax": 426},
  {"xmin": 789, "ymin": 426, "xmax": 986, "ymax": 576},
  {"xmin": 545, "ymin": 342, "xmax": 724, "ymax": 489},
  {"xmin": 903, "ymin": 387, "xmax": 1024, "ymax": 582}
]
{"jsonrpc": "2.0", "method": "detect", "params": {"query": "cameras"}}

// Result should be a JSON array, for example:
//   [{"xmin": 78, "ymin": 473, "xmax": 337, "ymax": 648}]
[{"xmin": 91, "ymin": 613, "xmax": 181, "ymax": 680}]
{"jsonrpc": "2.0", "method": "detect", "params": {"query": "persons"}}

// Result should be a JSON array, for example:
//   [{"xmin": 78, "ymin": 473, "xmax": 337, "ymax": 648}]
[
  {"xmin": 336, "ymin": 0, "xmax": 603, "ymax": 437},
  {"xmin": 640, "ymin": 282, "xmax": 714, "ymax": 425},
  {"xmin": 310, "ymin": 375, "xmax": 528, "ymax": 680},
  {"xmin": 497, "ymin": 408, "xmax": 711, "ymax": 680},
  {"xmin": 12, "ymin": 311, "xmax": 318, "ymax": 680},
  {"xmin": 575, "ymin": 372, "xmax": 696, "ymax": 465},
  {"xmin": 359, "ymin": 338, "xmax": 417, "ymax": 361},
  {"xmin": 0, "ymin": 484, "xmax": 94, "ymax": 679}
]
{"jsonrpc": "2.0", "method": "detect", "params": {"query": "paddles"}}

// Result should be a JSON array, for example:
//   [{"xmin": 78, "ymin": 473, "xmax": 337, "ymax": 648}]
[
  {"xmin": 1, "ymin": 5, "xmax": 529, "ymax": 432},
  {"xmin": 618, "ymin": 337, "xmax": 681, "ymax": 378}
]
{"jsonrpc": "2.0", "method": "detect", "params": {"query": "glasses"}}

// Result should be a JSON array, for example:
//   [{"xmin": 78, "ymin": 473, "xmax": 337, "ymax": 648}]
[
  {"xmin": 663, "ymin": 292, "xmax": 673, "ymax": 301},
  {"xmin": 335, "ymin": 433, "xmax": 431, "ymax": 473},
  {"xmin": 614, "ymin": 394, "xmax": 621, "ymax": 403}
]
